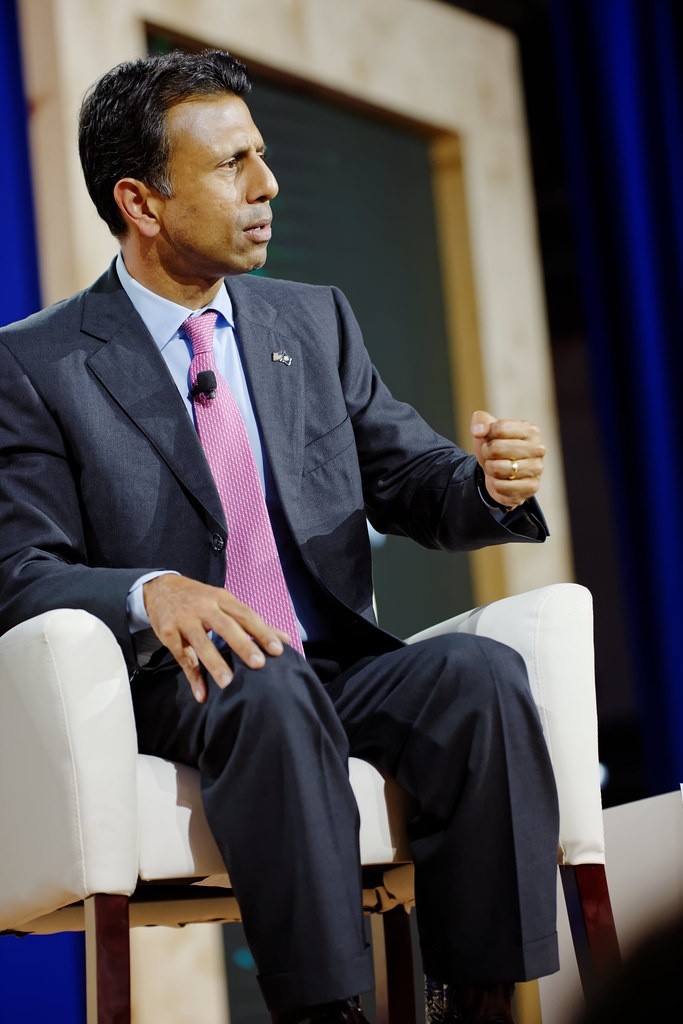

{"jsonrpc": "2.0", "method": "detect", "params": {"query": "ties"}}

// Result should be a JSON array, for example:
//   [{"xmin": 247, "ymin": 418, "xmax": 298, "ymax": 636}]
[{"xmin": 181, "ymin": 309, "xmax": 306, "ymax": 660}]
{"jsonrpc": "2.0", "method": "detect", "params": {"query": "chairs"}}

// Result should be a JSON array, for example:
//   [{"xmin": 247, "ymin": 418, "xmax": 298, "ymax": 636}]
[{"xmin": 0, "ymin": 582, "xmax": 638, "ymax": 1024}]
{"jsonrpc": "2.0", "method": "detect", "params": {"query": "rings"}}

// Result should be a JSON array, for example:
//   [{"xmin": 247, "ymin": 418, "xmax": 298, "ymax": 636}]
[{"xmin": 509, "ymin": 460, "xmax": 518, "ymax": 480}]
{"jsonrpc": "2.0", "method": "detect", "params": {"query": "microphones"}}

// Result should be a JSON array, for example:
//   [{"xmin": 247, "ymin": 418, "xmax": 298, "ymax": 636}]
[{"xmin": 196, "ymin": 371, "xmax": 216, "ymax": 400}]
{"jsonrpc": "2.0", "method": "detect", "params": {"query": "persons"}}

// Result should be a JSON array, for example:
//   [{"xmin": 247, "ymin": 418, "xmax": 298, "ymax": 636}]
[{"xmin": 1, "ymin": 50, "xmax": 561, "ymax": 1024}]
